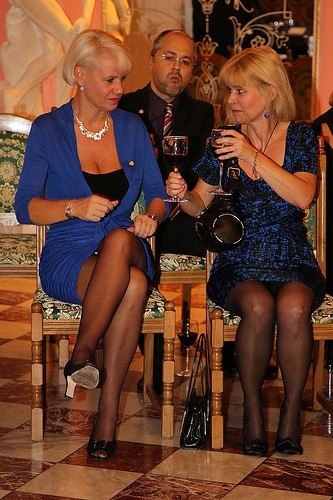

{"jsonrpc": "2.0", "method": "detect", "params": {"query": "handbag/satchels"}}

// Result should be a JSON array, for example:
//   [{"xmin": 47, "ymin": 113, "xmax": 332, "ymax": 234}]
[
  {"xmin": 180, "ymin": 333, "xmax": 211, "ymax": 450},
  {"xmin": 195, "ymin": 126, "xmax": 245, "ymax": 253}
]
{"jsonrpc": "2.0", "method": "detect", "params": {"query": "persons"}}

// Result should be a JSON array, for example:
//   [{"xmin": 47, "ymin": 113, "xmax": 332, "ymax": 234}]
[
  {"xmin": 118, "ymin": 29, "xmax": 213, "ymax": 395},
  {"xmin": 167, "ymin": 47, "xmax": 325, "ymax": 459},
  {"xmin": 0, "ymin": 0, "xmax": 130, "ymax": 121},
  {"xmin": 12, "ymin": 29, "xmax": 173, "ymax": 461}
]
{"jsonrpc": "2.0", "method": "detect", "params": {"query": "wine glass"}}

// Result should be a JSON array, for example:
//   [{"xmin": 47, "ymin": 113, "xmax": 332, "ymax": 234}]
[
  {"xmin": 175, "ymin": 319, "xmax": 200, "ymax": 377},
  {"xmin": 208, "ymin": 128, "xmax": 231, "ymax": 197}
]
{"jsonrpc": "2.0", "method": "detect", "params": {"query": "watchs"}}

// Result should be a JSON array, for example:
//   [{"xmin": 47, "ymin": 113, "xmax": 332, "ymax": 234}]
[{"xmin": 144, "ymin": 212, "xmax": 159, "ymax": 227}]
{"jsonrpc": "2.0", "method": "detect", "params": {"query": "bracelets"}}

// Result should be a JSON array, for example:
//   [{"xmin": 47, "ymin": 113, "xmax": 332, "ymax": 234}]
[
  {"xmin": 65, "ymin": 198, "xmax": 76, "ymax": 219},
  {"xmin": 252, "ymin": 150, "xmax": 259, "ymax": 179}
]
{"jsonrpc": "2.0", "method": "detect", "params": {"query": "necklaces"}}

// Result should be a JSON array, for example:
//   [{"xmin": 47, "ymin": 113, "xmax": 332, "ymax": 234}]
[
  {"xmin": 247, "ymin": 120, "xmax": 280, "ymax": 155},
  {"xmin": 71, "ymin": 104, "xmax": 110, "ymax": 141}
]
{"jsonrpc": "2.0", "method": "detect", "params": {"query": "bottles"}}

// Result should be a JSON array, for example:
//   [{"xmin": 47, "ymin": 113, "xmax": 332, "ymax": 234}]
[{"xmin": 160, "ymin": 135, "xmax": 190, "ymax": 204}]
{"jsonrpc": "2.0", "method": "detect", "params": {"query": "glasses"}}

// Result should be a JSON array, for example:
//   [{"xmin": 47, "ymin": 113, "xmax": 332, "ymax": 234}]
[{"xmin": 154, "ymin": 53, "xmax": 194, "ymax": 68}]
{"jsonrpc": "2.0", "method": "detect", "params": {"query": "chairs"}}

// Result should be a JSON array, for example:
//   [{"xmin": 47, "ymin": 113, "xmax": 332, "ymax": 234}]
[{"xmin": 0, "ymin": 111, "xmax": 333, "ymax": 449}]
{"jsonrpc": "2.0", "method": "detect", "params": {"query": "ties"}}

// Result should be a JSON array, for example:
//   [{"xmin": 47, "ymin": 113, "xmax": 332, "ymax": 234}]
[{"xmin": 161, "ymin": 103, "xmax": 175, "ymax": 156}]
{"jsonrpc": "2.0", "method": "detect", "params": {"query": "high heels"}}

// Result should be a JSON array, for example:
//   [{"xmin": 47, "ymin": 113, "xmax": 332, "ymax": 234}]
[
  {"xmin": 242, "ymin": 410, "xmax": 269, "ymax": 455},
  {"xmin": 63, "ymin": 360, "xmax": 100, "ymax": 404},
  {"xmin": 276, "ymin": 400, "xmax": 304, "ymax": 455},
  {"xmin": 87, "ymin": 412, "xmax": 117, "ymax": 460}
]
